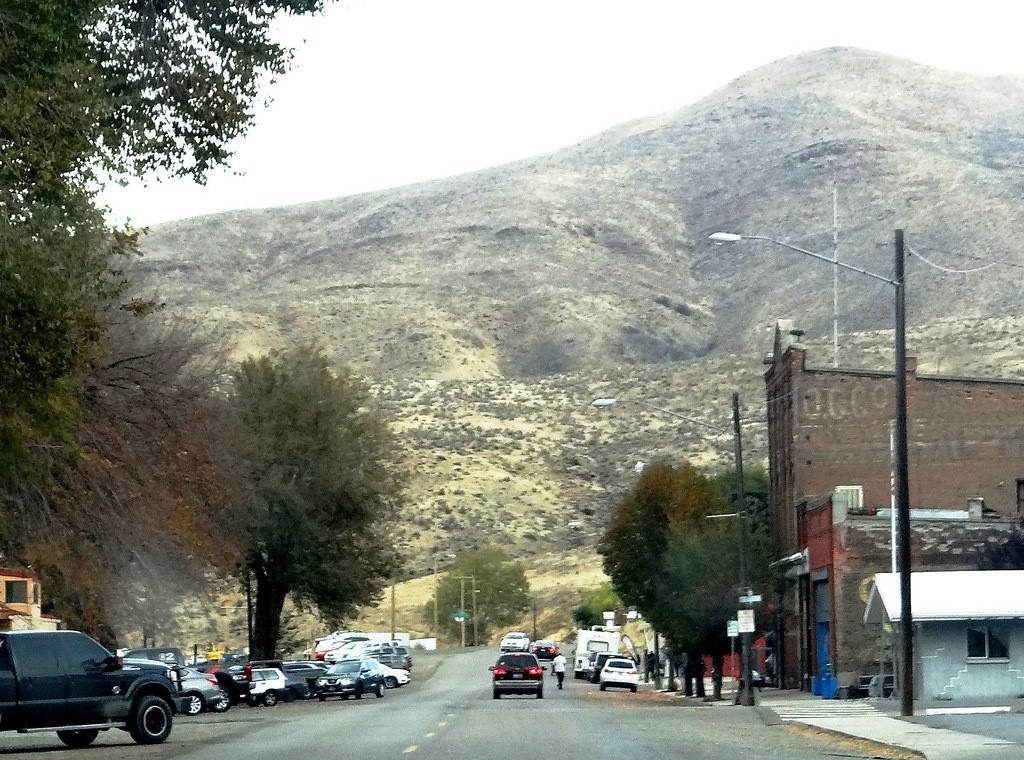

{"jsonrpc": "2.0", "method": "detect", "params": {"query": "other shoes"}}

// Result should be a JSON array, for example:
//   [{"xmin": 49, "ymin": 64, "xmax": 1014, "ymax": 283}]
[{"xmin": 557, "ymin": 684, "xmax": 562, "ymax": 690}]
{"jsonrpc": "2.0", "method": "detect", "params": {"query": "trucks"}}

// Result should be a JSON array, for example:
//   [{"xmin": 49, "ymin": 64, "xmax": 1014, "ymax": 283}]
[{"xmin": 573, "ymin": 624, "xmax": 625, "ymax": 681}]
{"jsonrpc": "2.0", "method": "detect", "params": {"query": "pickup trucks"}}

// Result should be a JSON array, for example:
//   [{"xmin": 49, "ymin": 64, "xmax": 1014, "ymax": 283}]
[{"xmin": 1, "ymin": 630, "xmax": 187, "ymax": 748}]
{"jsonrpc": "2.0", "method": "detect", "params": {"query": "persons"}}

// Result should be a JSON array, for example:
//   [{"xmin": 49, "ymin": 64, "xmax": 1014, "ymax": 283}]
[{"xmin": 553, "ymin": 652, "xmax": 567, "ymax": 690}]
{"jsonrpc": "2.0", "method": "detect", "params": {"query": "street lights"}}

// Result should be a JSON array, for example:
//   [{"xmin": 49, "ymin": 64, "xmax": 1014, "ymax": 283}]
[
  {"xmin": 709, "ymin": 230, "xmax": 913, "ymax": 717},
  {"xmin": 591, "ymin": 393, "xmax": 757, "ymax": 707},
  {"xmin": 432, "ymin": 545, "xmax": 474, "ymax": 637}
]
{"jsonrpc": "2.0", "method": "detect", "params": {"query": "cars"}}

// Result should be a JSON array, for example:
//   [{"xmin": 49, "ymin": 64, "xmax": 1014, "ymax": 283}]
[
  {"xmin": 490, "ymin": 652, "xmax": 548, "ymax": 700},
  {"xmin": 589, "ymin": 650, "xmax": 642, "ymax": 693},
  {"xmin": 529, "ymin": 637, "xmax": 559, "ymax": 661},
  {"xmin": 500, "ymin": 631, "xmax": 531, "ymax": 653},
  {"xmin": 121, "ymin": 633, "xmax": 414, "ymax": 717}
]
